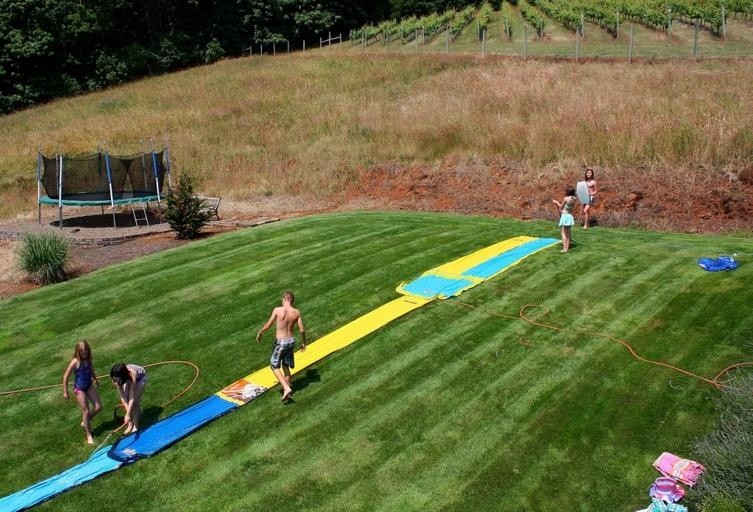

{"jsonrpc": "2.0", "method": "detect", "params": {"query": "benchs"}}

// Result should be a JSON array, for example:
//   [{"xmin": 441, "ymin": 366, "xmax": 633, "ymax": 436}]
[{"xmin": 191, "ymin": 195, "xmax": 221, "ymax": 221}]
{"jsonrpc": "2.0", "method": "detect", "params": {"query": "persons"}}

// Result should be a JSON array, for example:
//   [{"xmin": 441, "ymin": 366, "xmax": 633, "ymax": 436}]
[
  {"xmin": 63, "ymin": 340, "xmax": 103, "ymax": 445},
  {"xmin": 552, "ymin": 185, "xmax": 576, "ymax": 253},
  {"xmin": 255, "ymin": 291, "xmax": 306, "ymax": 401},
  {"xmin": 576, "ymin": 168, "xmax": 598, "ymax": 230},
  {"xmin": 110, "ymin": 361, "xmax": 147, "ymax": 435}
]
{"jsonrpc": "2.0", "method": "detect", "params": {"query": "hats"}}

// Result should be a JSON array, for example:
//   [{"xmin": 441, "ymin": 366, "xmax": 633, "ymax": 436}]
[{"xmin": 649, "ymin": 477, "xmax": 686, "ymax": 504}]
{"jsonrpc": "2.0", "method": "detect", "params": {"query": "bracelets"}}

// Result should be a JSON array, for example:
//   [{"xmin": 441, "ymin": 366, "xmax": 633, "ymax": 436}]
[{"xmin": 63, "ymin": 389, "xmax": 68, "ymax": 393}]
